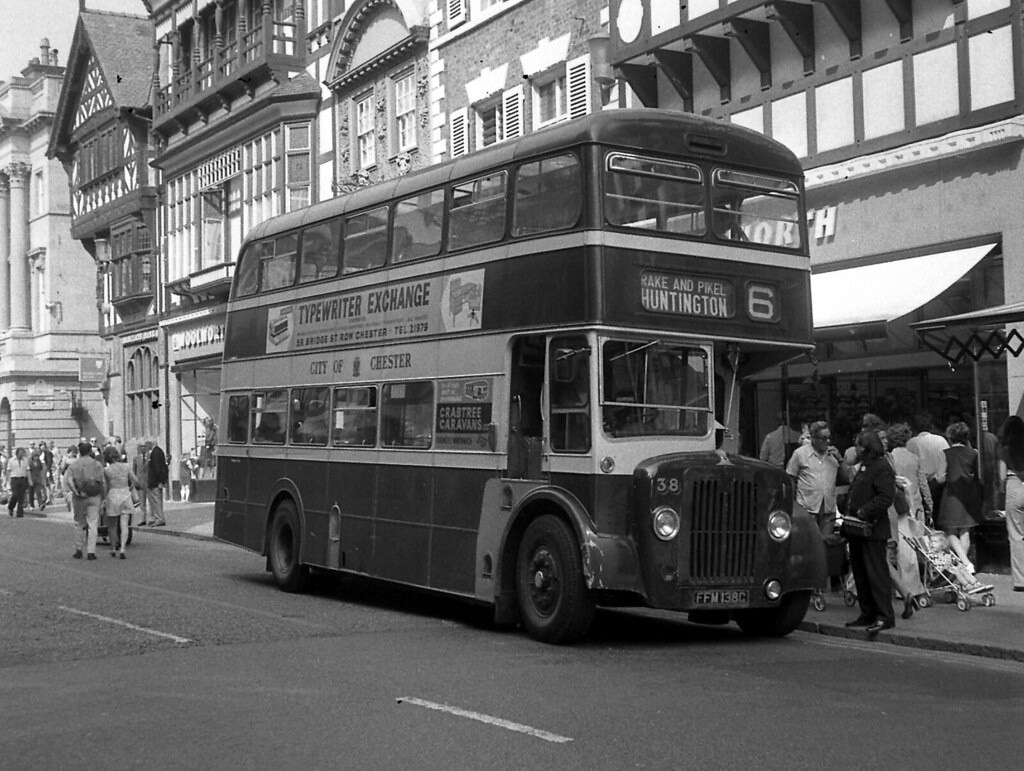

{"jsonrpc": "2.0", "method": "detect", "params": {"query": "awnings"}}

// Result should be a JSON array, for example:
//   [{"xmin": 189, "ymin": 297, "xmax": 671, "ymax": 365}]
[{"xmin": 812, "ymin": 242, "xmax": 996, "ymax": 339}]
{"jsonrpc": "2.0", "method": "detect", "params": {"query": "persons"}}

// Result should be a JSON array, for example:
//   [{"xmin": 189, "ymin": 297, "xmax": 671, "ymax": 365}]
[
  {"xmin": 0, "ymin": 437, "xmax": 169, "ymax": 560},
  {"xmin": 179, "ymin": 451, "xmax": 199, "ymax": 502},
  {"xmin": 759, "ymin": 409, "xmax": 1024, "ymax": 632}
]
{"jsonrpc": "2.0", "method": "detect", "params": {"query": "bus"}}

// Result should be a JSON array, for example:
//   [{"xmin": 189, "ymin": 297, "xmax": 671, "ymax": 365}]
[{"xmin": 211, "ymin": 107, "xmax": 829, "ymax": 644}]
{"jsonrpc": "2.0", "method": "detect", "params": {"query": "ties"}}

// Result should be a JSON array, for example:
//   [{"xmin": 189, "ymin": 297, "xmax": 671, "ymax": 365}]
[{"xmin": 143, "ymin": 453, "xmax": 146, "ymax": 465}]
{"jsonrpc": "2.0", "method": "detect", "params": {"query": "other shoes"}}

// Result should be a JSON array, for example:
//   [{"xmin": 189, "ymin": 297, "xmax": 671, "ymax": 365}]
[
  {"xmin": 17, "ymin": 515, "xmax": 24, "ymax": 517},
  {"xmin": 137, "ymin": 522, "xmax": 147, "ymax": 526},
  {"xmin": 40, "ymin": 504, "xmax": 45, "ymax": 511},
  {"xmin": 120, "ymin": 550, "xmax": 127, "ymax": 559},
  {"xmin": 866, "ymin": 620, "xmax": 890, "ymax": 632},
  {"xmin": 845, "ymin": 615, "xmax": 875, "ymax": 627},
  {"xmin": 8, "ymin": 507, "xmax": 13, "ymax": 516},
  {"xmin": 149, "ymin": 520, "xmax": 165, "ymax": 527},
  {"xmin": 110, "ymin": 549, "xmax": 116, "ymax": 558},
  {"xmin": 967, "ymin": 563, "xmax": 976, "ymax": 575},
  {"xmin": 30, "ymin": 506, "xmax": 34, "ymax": 511},
  {"xmin": 73, "ymin": 549, "xmax": 82, "ymax": 559},
  {"xmin": 87, "ymin": 553, "xmax": 97, "ymax": 560}
]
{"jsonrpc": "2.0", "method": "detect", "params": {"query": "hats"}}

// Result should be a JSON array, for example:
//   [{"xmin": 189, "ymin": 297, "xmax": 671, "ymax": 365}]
[{"xmin": 31, "ymin": 451, "xmax": 42, "ymax": 460}]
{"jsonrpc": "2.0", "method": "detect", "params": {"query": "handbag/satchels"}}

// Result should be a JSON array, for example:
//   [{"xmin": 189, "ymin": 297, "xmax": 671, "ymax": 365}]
[
  {"xmin": 960, "ymin": 479, "xmax": 985, "ymax": 523},
  {"xmin": 85, "ymin": 479, "xmax": 101, "ymax": 496},
  {"xmin": 840, "ymin": 516, "xmax": 872, "ymax": 538},
  {"xmin": 893, "ymin": 485, "xmax": 909, "ymax": 515}
]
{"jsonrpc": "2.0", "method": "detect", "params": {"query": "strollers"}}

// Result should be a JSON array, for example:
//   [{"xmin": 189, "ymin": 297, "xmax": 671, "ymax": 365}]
[
  {"xmin": 808, "ymin": 514, "xmax": 857, "ymax": 610},
  {"xmin": 893, "ymin": 515, "xmax": 998, "ymax": 610}
]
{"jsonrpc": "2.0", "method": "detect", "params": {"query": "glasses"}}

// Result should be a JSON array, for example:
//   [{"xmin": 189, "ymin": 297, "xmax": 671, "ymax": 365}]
[{"xmin": 815, "ymin": 435, "xmax": 833, "ymax": 442}]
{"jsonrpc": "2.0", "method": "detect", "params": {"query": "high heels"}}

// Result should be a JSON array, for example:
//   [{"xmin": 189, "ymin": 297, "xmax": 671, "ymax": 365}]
[{"xmin": 901, "ymin": 597, "xmax": 921, "ymax": 619}]
